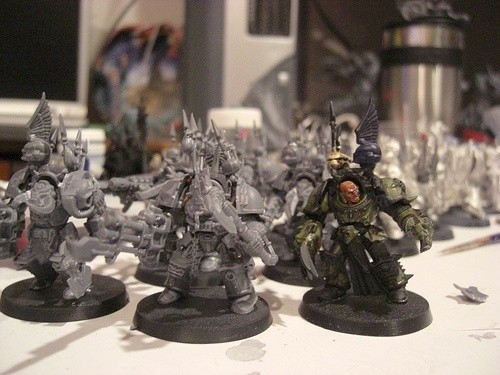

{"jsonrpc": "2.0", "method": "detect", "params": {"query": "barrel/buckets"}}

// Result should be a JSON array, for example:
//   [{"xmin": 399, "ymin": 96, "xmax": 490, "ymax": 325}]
[{"xmin": 382, "ymin": 10, "xmax": 464, "ymax": 134}]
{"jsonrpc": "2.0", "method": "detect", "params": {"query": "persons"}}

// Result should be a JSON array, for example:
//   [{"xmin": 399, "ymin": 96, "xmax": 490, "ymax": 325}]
[
  {"xmin": 294, "ymin": 138, "xmax": 437, "ymax": 305},
  {"xmin": 0, "ymin": 130, "xmax": 126, "ymax": 301},
  {"xmin": 137, "ymin": 163, "xmax": 280, "ymax": 317},
  {"xmin": 89, "ymin": 101, "xmax": 500, "ymax": 271}
]
